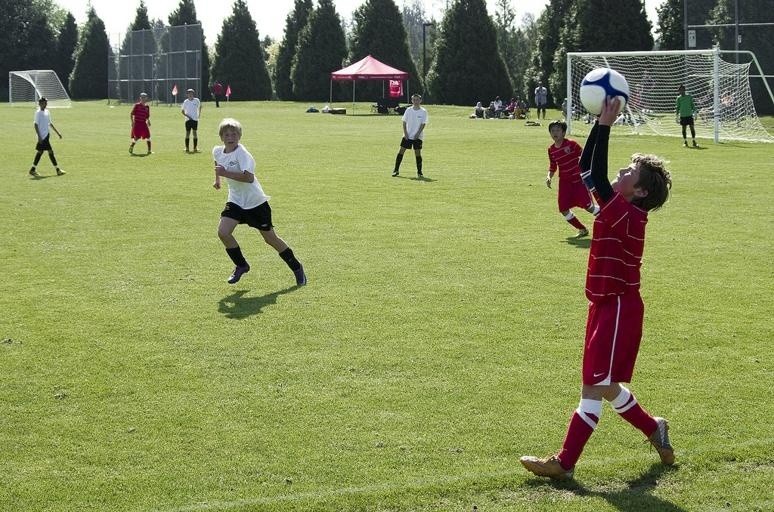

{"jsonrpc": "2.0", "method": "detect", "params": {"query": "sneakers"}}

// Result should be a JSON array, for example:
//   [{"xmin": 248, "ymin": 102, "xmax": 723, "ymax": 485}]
[
  {"xmin": 228, "ymin": 264, "xmax": 250, "ymax": 284},
  {"xmin": 392, "ymin": 168, "xmax": 399, "ymax": 176},
  {"xmin": 57, "ymin": 170, "xmax": 66, "ymax": 175},
  {"xmin": 520, "ymin": 455, "xmax": 575, "ymax": 482},
  {"xmin": 643, "ymin": 417, "xmax": 675, "ymax": 465},
  {"xmin": 577, "ymin": 229, "xmax": 589, "ymax": 237},
  {"xmin": 128, "ymin": 148, "xmax": 153, "ymax": 154},
  {"xmin": 294, "ymin": 264, "xmax": 306, "ymax": 285},
  {"xmin": 184, "ymin": 148, "xmax": 200, "ymax": 154},
  {"xmin": 29, "ymin": 170, "xmax": 39, "ymax": 176}
]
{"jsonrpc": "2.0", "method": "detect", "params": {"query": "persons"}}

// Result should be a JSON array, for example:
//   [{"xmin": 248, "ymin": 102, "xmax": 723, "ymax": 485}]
[
  {"xmin": 29, "ymin": 97, "xmax": 66, "ymax": 176},
  {"xmin": 472, "ymin": 95, "xmax": 530, "ymax": 120},
  {"xmin": 392, "ymin": 94, "xmax": 428, "ymax": 177},
  {"xmin": 561, "ymin": 69, "xmax": 654, "ymax": 126},
  {"xmin": 213, "ymin": 117, "xmax": 307, "ymax": 285},
  {"xmin": 129, "ymin": 92, "xmax": 154, "ymax": 154},
  {"xmin": 674, "ymin": 85, "xmax": 697, "ymax": 147},
  {"xmin": 213, "ymin": 81, "xmax": 222, "ymax": 107},
  {"xmin": 182, "ymin": 89, "xmax": 201, "ymax": 151},
  {"xmin": 535, "ymin": 81, "xmax": 548, "ymax": 119},
  {"xmin": 546, "ymin": 120, "xmax": 602, "ymax": 238},
  {"xmin": 520, "ymin": 94, "xmax": 676, "ymax": 480}
]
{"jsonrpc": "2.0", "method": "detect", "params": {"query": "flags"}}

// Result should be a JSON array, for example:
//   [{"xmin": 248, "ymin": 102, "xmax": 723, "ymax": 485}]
[
  {"xmin": 172, "ymin": 85, "xmax": 178, "ymax": 95},
  {"xmin": 225, "ymin": 84, "xmax": 232, "ymax": 96}
]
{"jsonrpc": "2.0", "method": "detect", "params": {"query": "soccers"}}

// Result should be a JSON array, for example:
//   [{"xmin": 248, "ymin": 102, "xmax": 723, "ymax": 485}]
[{"xmin": 580, "ymin": 68, "xmax": 628, "ymax": 114}]
